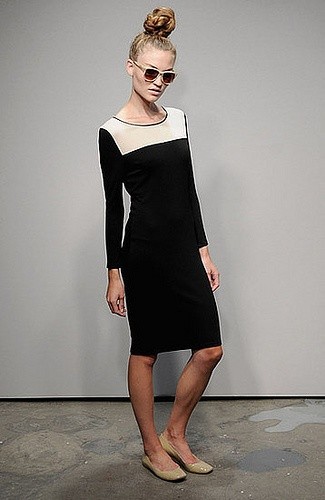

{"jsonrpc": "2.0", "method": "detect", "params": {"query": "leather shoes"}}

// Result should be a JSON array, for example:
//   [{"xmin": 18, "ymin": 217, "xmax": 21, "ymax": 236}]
[
  {"xmin": 142, "ymin": 454, "xmax": 186, "ymax": 480},
  {"xmin": 159, "ymin": 433, "xmax": 212, "ymax": 474}
]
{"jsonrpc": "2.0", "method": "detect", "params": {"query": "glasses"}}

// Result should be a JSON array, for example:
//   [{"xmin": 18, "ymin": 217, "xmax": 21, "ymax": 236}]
[{"xmin": 133, "ymin": 61, "xmax": 181, "ymax": 85}]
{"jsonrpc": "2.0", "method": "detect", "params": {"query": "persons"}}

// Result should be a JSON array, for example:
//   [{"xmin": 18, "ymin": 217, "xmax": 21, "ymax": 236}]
[{"xmin": 95, "ymin": 6, "xmax": 226, "ymax": 484}]
